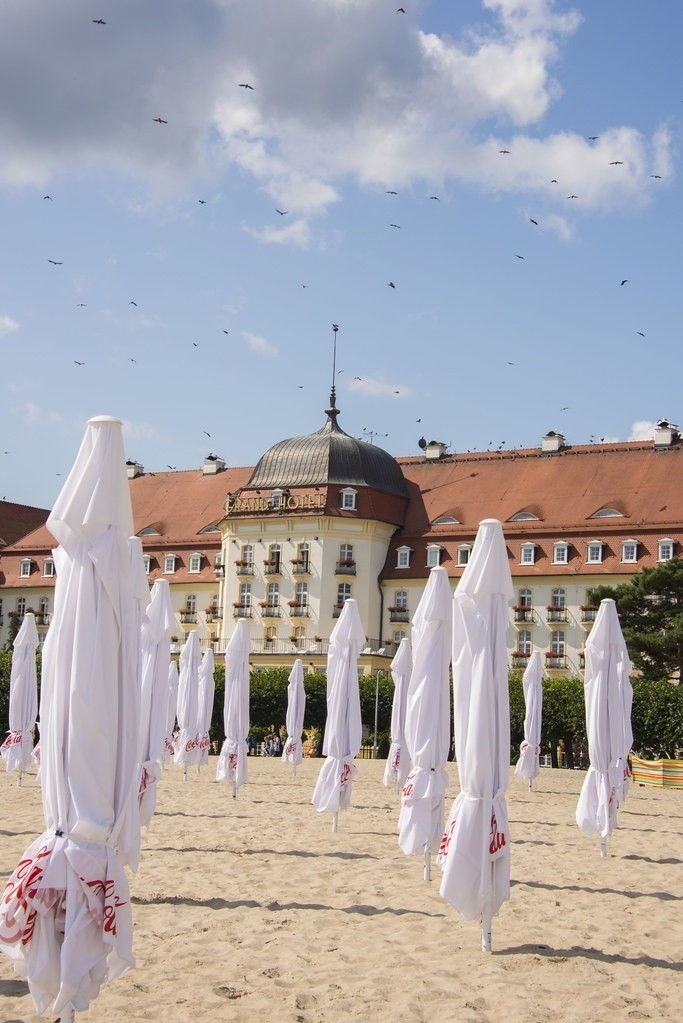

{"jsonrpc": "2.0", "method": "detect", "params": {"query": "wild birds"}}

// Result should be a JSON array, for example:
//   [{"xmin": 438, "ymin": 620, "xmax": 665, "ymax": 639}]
[{"xmin": 44, "ymin": 9, "xmax": 663, "ymax": 478}]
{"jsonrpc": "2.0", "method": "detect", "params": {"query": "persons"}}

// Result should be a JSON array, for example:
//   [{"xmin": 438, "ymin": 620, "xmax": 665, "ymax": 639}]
[
  {"xmin": 245, "ymin": 734, "xmax": 257, "ymax": 756},
  {"xmin": 262, "ymin": 732, "xmax": 281, "ymax": 758}
]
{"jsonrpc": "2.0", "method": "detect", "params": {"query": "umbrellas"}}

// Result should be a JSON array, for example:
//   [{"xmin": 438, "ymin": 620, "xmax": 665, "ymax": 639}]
[
  {"xmin": 0, "ymin": 613, "xmax": 40, "ymax": 793},
  {"xmin": 310, "ymin": 599, "xmax": 366, "ymax": 832},
  {"xmin": 126, "ymin": 535, "xmax": 152, "ymax": 638},
  {"xmin": 513, "ymin": 651, "xmax": 545, "ymax": 793},
  {"xmin": 575, "ymin": 599, "xmax": 633, "ymax": 858},
  {"xmin": 436, "ymin": 519, "xmax": 516, "ymax": 958},
  {"xmin": 176, "ymin": 630, "xmax": 199, "ymax": 782},
  {"xmin": 156, "ymin": 661, "xmax": 178, "ymax": 773},
  {"xmin": 214, "ymin": 617, "xmax": 251, "ymax": 799},
  {"xmin": 397, "ymin": 565, "xmax": 454, "ymax": 883},
  {"xmin": 382, "ymin": 637, "xmax": 415, "ymax": 796},
  {"xmin": 0, "ymin": 417, "xmax": 143, "ymax": 1023},
  {"xmin": 193, "ymin": 649, "xmax": 215, "ymax": 774},
  {"xmin": 281, "ymin": 659, "xmax": 306, "ymax": 777},
  {"xmin": 138, "ymin": 578, "xmax": 184, "ymax": 832}
]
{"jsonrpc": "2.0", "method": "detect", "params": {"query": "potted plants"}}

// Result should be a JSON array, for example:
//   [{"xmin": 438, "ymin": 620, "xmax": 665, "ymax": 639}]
[
  {"xmin": 232, "ymin": 600, "xmax": 244, "ymax": 609},
  {"xmin": 209, "ymin": 634, "xmax": 221, "ymax": 643},
  {"xmin": 212, "ymin": 561, "xmax": 222, "ymax": 571},
  {"xmin": 544, "ymin": 603, "xmax": 564, "ymax": 613},
  {"xmin": 204, "ymin": 603, "xmax": 217, "ymax": 616},
  {"xmin": 339, "ymin": 558, "xmax": 357, "ymax": 567},
  {"xmin": 545, "ymin": 651, "xmax": 564, "ymax": 658},
  {"xmin": 288, "ymin": 634, "xmax": 298, "ymax": 643},
  {"xmin": 286, "ymin": 599, "xmax": 301, "ymax": 608},
  {"xmin": 313, "ymin": 633, "xmax": 323, "ymax": 643},
  {"xmin": 233, "ymin": 558, "xmax": 248, "ymax": 567},
  {"xmin": 33, "ymin": 608, "xmax": 45, "ymax": 617},
  {"xmin": 510, "ymin": 650, "xmax": 531, "ymax": 658},
  {"xmin": 263, "ymin": 633, "xmax": 274, "ymax": 647},
  {"xmin": 579, "ymin": 602, "xmax": 599, "ymax": 612},
  {"xmin": 257, "ymin": 600, "xmax": 278, "ymax": 609},
  {"xmin": 176, "ymin": 606, "xmax": 196, "ymax": 616},
  {"xmin": 512, "ymin": 605, "xmax": 533, "ymax": 613},
  {"xmin": 170, "ymin": 635, "xmax": 179, "ymax": 643},
  {"xmin": 262, "ymin": 559, "xmax": 277, "ymax": 566},
  {"xmin": 384, "ymin": 637, "xmax": 393, "ymax": 645},
  {"xmin": 386, "ymin": 605, "xmax": 409, "ymax": 614},
  {"xmin": 289, "ymin": 558, "xmax": 304, "ymax": 566},
  {"xmin": 336, "ymin": 601, "xmax": 345, "ymax": 608}
]
